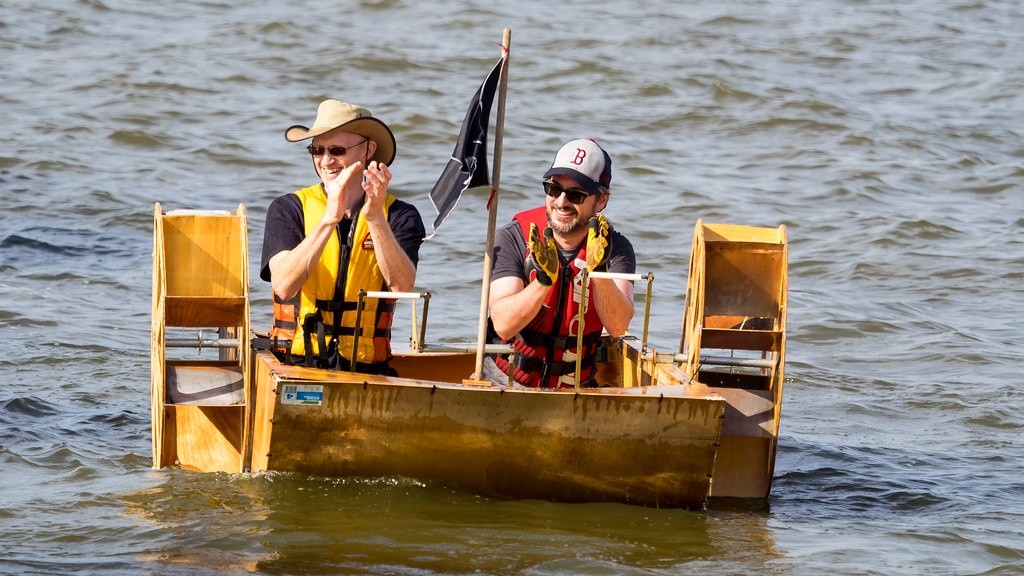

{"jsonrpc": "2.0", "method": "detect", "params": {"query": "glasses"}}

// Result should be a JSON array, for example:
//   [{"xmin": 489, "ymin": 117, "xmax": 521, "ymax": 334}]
[
  {"xmin": 543, "ymin": 181, "xmax": 589, "ymax": 204},
  {"xmin": 306, "ymin": 140, "xmax": 367, "ymax": 156}
]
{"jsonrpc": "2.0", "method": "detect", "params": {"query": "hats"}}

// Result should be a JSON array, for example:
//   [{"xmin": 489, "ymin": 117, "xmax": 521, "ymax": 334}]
[
  {"xmin": 284, "ymin": 99, "xmax": 396, "ymax": 168},
  {"xmin": 543, "ymin": 138, "xmax": 612, "ymax": 190}
]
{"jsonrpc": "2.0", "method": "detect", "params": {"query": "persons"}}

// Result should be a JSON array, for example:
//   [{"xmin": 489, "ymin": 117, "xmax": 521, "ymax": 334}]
[
  {"xmin": 260, "ymin": 100, "xmax": 426, "ymax": 378},
  {"xmin": 481, "ymin": 139, "xmax": 636, "ymax": 388}
]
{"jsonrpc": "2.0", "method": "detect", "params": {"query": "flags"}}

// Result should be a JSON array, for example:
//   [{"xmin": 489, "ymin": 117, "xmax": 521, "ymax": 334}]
[{"xmin": 422, "ymin": 49, "xmax": 506, "ymax": 242}]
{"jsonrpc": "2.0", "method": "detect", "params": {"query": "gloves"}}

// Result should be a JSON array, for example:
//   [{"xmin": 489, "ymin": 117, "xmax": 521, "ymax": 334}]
[
  {"xmin": 528, "ymin": 223, "xmax": 559, "ymax": 287},
  {"xmin": 586, "ymin": 213, "xmax": 614, "ymax": 273}
]
